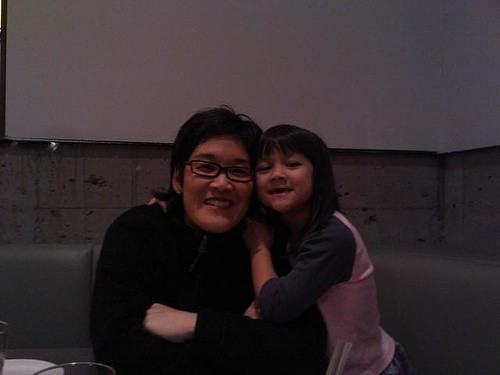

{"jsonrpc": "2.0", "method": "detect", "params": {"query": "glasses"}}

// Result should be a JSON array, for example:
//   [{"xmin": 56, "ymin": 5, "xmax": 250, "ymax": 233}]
[{"xmin": 182, "ymin": 160, "xmax": 257, "ymax": 184}]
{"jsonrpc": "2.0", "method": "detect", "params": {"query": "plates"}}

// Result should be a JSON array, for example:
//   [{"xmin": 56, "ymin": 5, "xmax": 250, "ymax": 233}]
[{"xmin": 2, "ymin": 359, "xmax": 64, "ymax": 375}]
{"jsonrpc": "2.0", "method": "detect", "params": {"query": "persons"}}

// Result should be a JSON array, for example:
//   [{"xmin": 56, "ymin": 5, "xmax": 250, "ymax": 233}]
[
  {"xmin": 90, "ymin": 107, "xmax": 328, "ymax": 375},
  {"xmin": 147, "ymin": 124, "xmax": 412, "ymax": 375}
]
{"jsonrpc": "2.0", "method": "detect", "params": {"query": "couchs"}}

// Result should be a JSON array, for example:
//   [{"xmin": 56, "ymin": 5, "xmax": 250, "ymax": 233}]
[{"xmin": 0, "ymin": 243, "xmax": 500, "ymax": 375}]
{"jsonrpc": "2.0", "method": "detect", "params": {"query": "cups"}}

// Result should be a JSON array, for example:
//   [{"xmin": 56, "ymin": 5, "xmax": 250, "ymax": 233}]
[
  {"xmin": 0, "ymin": 321, "xmax": 9, "ymax": 375},
  {"xmin": 33, "ymin": 362, "xmax": 115, "ymax": 375}
]
